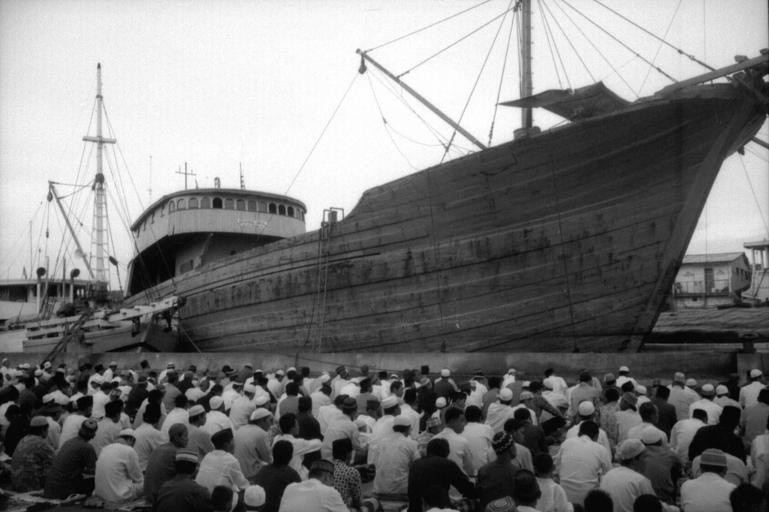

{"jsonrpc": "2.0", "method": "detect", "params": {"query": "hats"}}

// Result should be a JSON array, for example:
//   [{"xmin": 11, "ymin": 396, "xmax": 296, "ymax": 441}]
[
  {"xmin": 242, "ymin": 484, "xmax": 268, "ymax": 507},
  {"xmin": 617, "ymin": 426, "xmax": 661, "ymax": 460},
  {"xmin": 484, "ymin": 496, "xmax": 516, "ymax": 512},
  {"xmin": 340, "ymin": 397, "xmax": 357, "ymax": 409},
  {"xmin": 749, "ymin": 370, "xmax": 763, "ymax": 377},
  {"xmin": 105, "ymin": 400, "xmax": 122, "ymax": 410},
  {"xmin": 244, "ymin": 384, "xmax": 271, "ymax": 421},
  {"xmin": 491, "ymin": 432, "xmax": 514, "ymax": 453},
  {"xmin": 435, "ymin": 398, "xmax": 446, "ymax": 408},
  {"xmin": 518, "ymin": 390, "xmax": 534, "ymax": 401},
  {"xmin": 134, "ymin": 377, "xmax": 148, "ymax": 385},
  {"xmin": 146, "ymin": 389, "xmax": 166, "ymax": 416},
  {"xmin": 381, "ymin": 396, "xmax": 398, "ymax": 409},
  {"xmin": 205, "ymin": 365, "xmax": 235, "ymax": 379},
  {"xmin": 276, "ymin": 370, "xmax": 284, "ymax": 375},
  {"xmin": 211, "ymin": 428, "xmax": 235, "ymax": 442},
  {"xmin": 310, "ymin": 460, "xmax": 335, "ymax": 471},
  {"xmin": 604, "ymin": 365, "xmax": 646, "ymax": 407},
  {"xmin": 82, "ymin": 419, "xmax": 98, "ymax": 431},
  {"xmin": 188, "ymin": 404, "xmax": 207, "ymax": 415},
  {"xmin": 393, "ymin": 416, "xmax": 410, "ymax": 428},
  {"xmin": 332, "ymin": 440, "xmax": 352, "ymax": 454},
  {"xmin": 174, "ymin": 449, "xmax": 200, "ymax": 463},
  {"xmin": 441, "ymin": 368, "xmax": 450, "ymax": 378},
  {"xmin": 500, "ymin": 389, "xmax": 513, "ymax": 401},
  {"xmin": 29, "ymin": 415, "xmax": 48, "ymax": 427},
  {"xmin": 699, "ymin": 450, "xmax": 729, "ymax": 467},
  {"xmin": 207, "ymin": 395, "xmax": 224, "ymax": 409},
  {"xmin": 674, "ymin": 371, "xmax": 729, "ymax": 396},
  {"xmin": 118, "ymin": 427, "xmax": 136, "ymax": 439},
  {"xmin": 579, "ymin": 402, "xmax": 594, "ymax": 416},
  {"xmin": 77, "ymin": 394, "xmax": 94, "ymax": 407}
]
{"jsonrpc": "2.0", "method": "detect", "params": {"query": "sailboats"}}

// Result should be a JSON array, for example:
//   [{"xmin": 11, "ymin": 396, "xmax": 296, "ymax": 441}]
[{"xmin": 1, "ymin": 60, "xmax": 181, "ymax": 355}]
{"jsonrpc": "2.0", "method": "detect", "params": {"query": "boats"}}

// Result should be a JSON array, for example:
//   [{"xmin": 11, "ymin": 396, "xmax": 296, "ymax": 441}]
[{"xmin": 123, "ymin": 0, "xmax": 768, "ymax": 359}]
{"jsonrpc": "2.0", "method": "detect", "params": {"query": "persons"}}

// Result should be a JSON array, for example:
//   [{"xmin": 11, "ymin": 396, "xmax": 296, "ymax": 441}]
[{"xmin": 2, "ymin": 356, "xmax": 768, "ymax": 512}]
